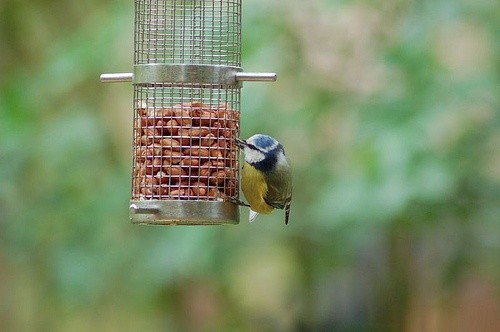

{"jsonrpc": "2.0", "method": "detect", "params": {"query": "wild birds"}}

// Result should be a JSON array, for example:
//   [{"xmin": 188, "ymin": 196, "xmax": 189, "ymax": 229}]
[{"xmin": 222, "ymin": 133, "xmax": 294, "ymax": 226}]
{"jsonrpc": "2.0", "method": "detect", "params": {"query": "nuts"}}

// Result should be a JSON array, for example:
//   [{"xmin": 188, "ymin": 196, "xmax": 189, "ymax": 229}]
[{"xmin": 134, "ymin": 100, "xmax": 240, "ymax": 202}]
